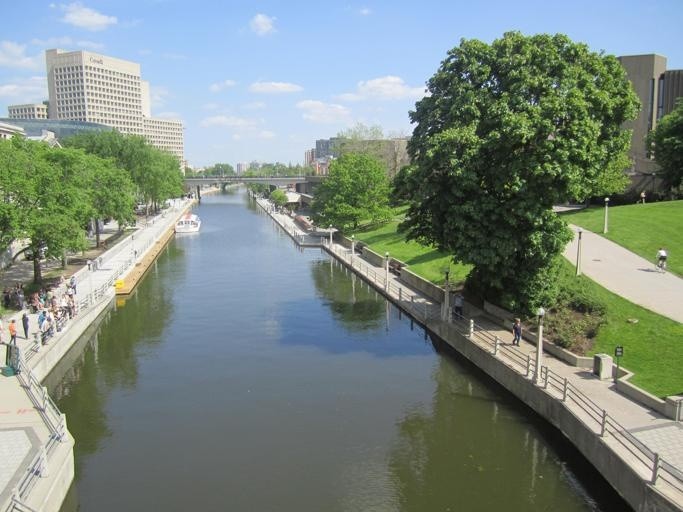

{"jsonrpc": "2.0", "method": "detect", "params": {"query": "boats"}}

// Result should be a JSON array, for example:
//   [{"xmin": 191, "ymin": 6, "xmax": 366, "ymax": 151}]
[{"xmin": 174, "ymin": 211, "xmax": 200, "ymax": 233}]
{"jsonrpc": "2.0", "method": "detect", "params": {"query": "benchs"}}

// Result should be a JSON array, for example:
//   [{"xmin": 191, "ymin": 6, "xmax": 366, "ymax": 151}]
[
  {"xmin": 354, "ymin": 241, "xmax": 369, "ymax": 253},
  {"xmin": 385, "ymin": 258, "xmax": 407, "ymax": 275}
]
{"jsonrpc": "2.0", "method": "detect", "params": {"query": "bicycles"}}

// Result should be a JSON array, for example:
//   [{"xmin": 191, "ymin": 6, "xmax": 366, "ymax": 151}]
[{"xmin": 654, "ymin": 256, "xmax": 668, "ymax": 273}]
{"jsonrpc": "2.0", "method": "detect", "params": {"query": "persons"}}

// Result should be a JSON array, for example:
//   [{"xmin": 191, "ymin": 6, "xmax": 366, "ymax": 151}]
[
  {"xmin": 656, "ymin": 248, "xmax": 668, "ymax": 268},
  {"xmin": 453, "ymin": 292, "xmax": 465, "ymax": 319},
  {"xmin": 0, "ymin": 259, "xmax": 92, "ymax": 346},
  {"xmin": 636, "ymin": 189, "xmax": 646, "ymax": 205},
  {"xmin": 512, "ymin": 318, "xmax": 522, "ymax": 347}
]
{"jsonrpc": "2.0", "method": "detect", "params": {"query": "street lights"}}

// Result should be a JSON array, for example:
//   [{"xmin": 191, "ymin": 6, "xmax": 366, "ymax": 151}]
[
  {"xmin": 384, "ymin": 252, "xmax": 389, "ymax": 288},
  {"xmin": 351, "ymin": 234, "xmax": 354, "ymax": 261},
  {"xmin": 535, "ymin": 307, "xmax": 545, "ymax": 378},
  {"xmin": 576, "ymin": 227, "xmax": 583, "ymax": 275},
  {"xmin": 329, "ymin": 224, "xmax": 332, "ymax": 248},
  {"xmin": 443, "ymin": 265, "xmax": 450, "ymax": 316},
  {"xmin": 603, "ymin": 197, "xmax": 609, "ymax": 234},
  {"xmin": 640, "ymin": 192, "xmax": 645, "ymax": 204}
]
